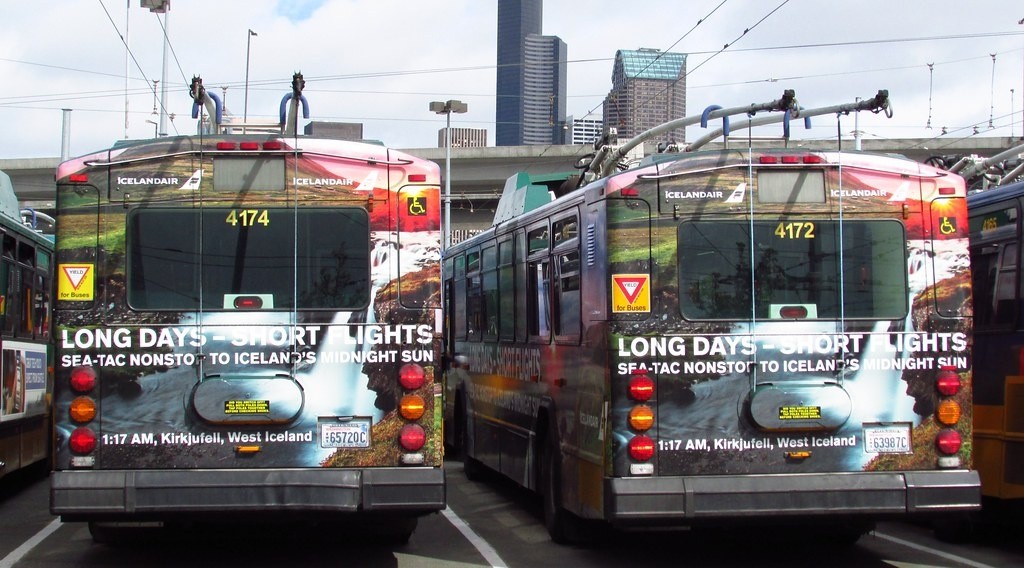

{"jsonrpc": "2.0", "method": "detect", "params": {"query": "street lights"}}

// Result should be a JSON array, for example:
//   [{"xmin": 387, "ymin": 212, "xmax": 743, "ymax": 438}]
[{"xmin": 429, "ymin": 99, "xmax": 468, "ymax": 250}]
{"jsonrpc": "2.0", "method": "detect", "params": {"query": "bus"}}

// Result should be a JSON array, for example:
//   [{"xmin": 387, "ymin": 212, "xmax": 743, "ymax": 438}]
[{"xmin": 0, "ymin": 72, "xmax": 1024, "ymax": 568}]
